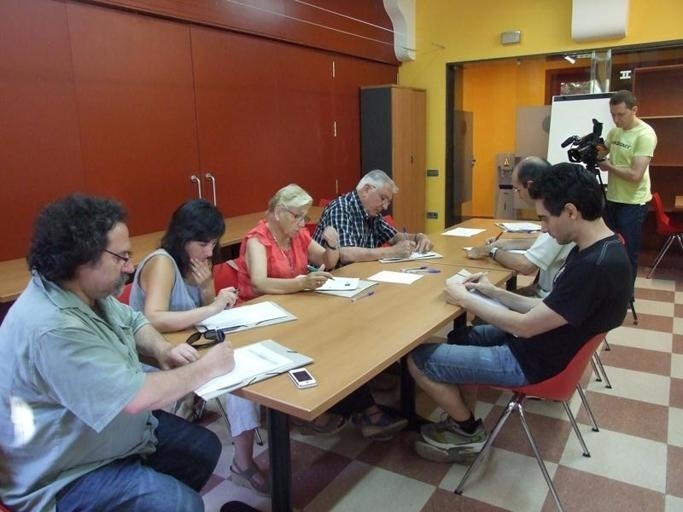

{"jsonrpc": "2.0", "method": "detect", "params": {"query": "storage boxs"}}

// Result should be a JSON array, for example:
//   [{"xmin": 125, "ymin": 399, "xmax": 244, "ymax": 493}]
[{"xmin": 675, "ymin": 195, "xmax": 683, "ymax": 208}]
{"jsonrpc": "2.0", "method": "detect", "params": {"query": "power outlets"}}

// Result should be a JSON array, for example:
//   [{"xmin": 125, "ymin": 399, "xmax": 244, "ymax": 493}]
[{"xmin": 427, "ymin": 212, "xmax": 438, "ymax": 220}]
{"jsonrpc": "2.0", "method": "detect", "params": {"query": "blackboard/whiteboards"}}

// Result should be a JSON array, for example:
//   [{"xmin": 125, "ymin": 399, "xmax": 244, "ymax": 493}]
[{"xmin": 547, "ymin": 94, "xmax": 615, "ymax": 185}]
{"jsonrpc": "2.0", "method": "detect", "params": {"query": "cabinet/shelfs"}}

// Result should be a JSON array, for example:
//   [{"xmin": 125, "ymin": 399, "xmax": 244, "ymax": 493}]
[
  {"xmin": 360, "ymin": 84, "xmax": 427, "ymax": 234},
  {"xmin": 452, "ymin": 113, "xmax": 475, "ymax": 205},
  {"xmin": 1, "ymin": 3, "xmax": 336, "ymax": 261},
  {"xmin": 632, "ymin": 64, "xmax": 683, "ymax": 267}
]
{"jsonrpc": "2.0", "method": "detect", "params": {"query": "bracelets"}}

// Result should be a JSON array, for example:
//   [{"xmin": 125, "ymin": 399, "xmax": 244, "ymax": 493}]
[{"xmin": 325, "ymin": 240, "xmax": 341, "ymax": 251}]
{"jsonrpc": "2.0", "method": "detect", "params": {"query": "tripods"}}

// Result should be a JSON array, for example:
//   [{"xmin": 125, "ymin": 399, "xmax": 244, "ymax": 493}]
[{"xmin": 587, "ymin": 169, "xmax": 639, "ymax": 325}]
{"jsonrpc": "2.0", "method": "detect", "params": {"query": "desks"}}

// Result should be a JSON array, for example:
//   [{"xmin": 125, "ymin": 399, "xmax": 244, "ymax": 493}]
[
  {"xmin": 340, "ymin": 216, "xmax": 542, "ymax": 292},
  {"xmin": 157, "ymin": 269, "xmax": 513, "ymax": 512},
  {"xmin": 0, "ymin": 199, "xmax": 324, "ymax": 307}
]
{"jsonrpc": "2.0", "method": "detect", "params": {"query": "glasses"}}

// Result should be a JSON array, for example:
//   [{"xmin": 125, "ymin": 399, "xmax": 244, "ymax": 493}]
[
  {"xmin": 281, "ymin": 207, "xmax": 311, "ymax": 223},
  {"xmin": 184, "ymin": 329, "xmax": 225, "ymax": 350}
]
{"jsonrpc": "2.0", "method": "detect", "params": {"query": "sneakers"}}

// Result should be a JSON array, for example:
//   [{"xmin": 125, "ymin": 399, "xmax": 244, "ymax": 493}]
[
  {"xmin": 419, "ymin": 415, "xmax": 488, "ymax": 454},
  {"xmin": 413, "ymin": 439, "xmax": 481, "ymax": 463}
]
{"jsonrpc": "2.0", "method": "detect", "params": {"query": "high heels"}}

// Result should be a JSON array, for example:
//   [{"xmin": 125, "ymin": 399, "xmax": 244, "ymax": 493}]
[
  {"xmin": 296, "ymin": 411, "xmax": 348, "ymax": 436},
  {"xmin": 358, "ymin": 410, "xmax": 409, "ymax": 442},
  {"xmin": 228, "ymin": 457, "xmax": 271, "ymax": 497}
]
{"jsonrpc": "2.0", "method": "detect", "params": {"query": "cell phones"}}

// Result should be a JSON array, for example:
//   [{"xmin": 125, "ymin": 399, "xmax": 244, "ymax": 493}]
[{"xmin": 286, "ymin": 367, "xmax": 318, "ymax": 390}]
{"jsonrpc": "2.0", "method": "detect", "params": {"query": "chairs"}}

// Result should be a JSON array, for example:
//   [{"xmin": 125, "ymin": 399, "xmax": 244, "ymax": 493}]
[
  {"xmin": 379, "ymin": 217, "xmax": 395, "ymax": 240},
  {"xmin": 451, "ymin": 332, "xmax": 607, "ymax": 512},
  {"xmin": 646, "ymin": 191, "xmax": 683, "ymax": 279},
  {"xmin": 116, "ymin": 285, "xmax": 271, "ymax": 446},
  {"xmin": 305, "ymin": 223, "xmax": 318, "ymax": 235},
  {"xmin": 210, "ymin": 259, "xmax": 236, "ymax": 293},
  {"xmin": 613, "ymin": 228, "xmax": 626, "ymax": 248}
]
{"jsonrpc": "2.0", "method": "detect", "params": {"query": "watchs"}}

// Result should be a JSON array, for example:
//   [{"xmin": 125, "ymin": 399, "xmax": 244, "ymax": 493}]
[{"xmin": 488, "ymin": 247, "xmax": 498, "ymax": 261}]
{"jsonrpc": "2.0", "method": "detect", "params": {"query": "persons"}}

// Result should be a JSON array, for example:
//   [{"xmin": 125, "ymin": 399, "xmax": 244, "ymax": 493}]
[
  {"xmin": 0, "ymin": 193, "xmax": 237, "ymax": 511},
  {"xmin": 467, "ymin": 154, "xmax": 577, "ymax": 327},
  {"xmin": 129, "ymin": 198, "xmax": 275, "ymax": 497},
  {"xmin": 598, "ymin": 87, "xmax": 658, "ymax": 314},
  {"xmin": 307, "ymin": 169, "xmax": 434, "ymax": 392},
  {"xmin": 404, "ymin": 159, "xmax": 634, "ymax": 463},
  {"xmin": 238, "ymin": 182, "xmax": 410, "ymax": 440}
]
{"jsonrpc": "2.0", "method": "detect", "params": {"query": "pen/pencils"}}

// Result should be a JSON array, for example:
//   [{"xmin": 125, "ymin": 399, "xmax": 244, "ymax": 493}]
[
  {"xmin": 407, "ymin": 269, "xmax": 440, "ymax": 273},
  {"xmin": 401, "ymin": 266, "xmax": 428, "ymax": 272},
  {"xmin": 215, "ymin": 327, "xmax": 223, "ymax": 343},
  {"xmin": 234, "ymin": 288, "xmax": 239, "ymax": 293},
  {"xmin": 404, "ymin": 226, "xmax": 408, "ymax": 240},
  {"xmin": 496, "ymin": 231, "xmax": 503, "ymax": 240},
  {"xmin": 307, "ymin": 265, "xmax": 335, "ymax": 281},
  {"xmin": 352, "ymin": 291, "xmax": 374, "ymax": 302},
  {"xmin": 470, "ymin": 271, "xmax": 488, "ymax": 282}
]
